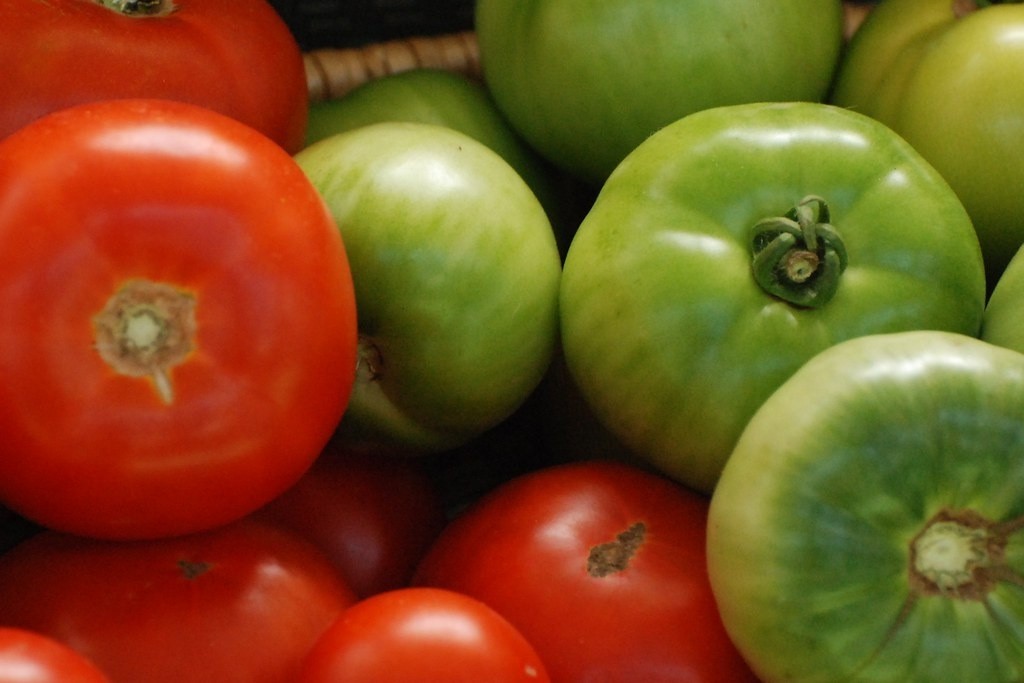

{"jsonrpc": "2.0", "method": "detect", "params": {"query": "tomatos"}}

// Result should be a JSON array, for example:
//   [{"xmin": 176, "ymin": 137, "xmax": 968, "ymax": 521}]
[{"xmin": 0, "ymin": 0, "xmax": 1024, "ymax": 683}]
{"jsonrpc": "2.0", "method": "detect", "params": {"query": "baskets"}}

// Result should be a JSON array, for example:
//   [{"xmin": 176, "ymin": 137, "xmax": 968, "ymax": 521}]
[{"xmin": 303, "ymin": 0, "xmax": 979, "ymax": 104}]
